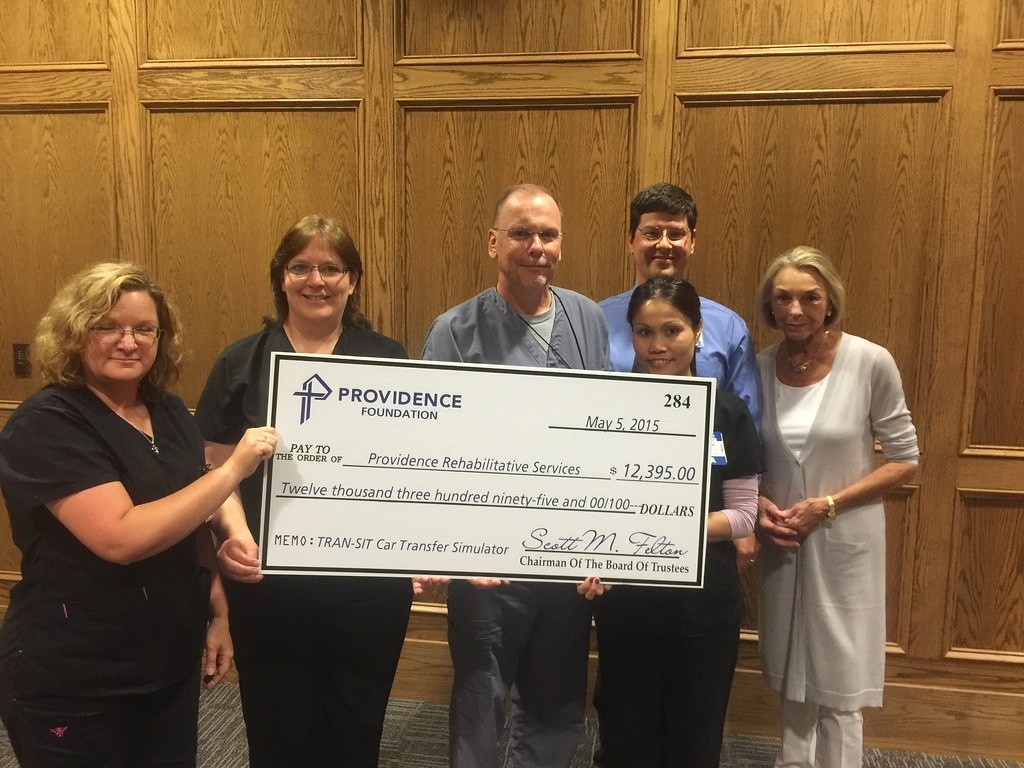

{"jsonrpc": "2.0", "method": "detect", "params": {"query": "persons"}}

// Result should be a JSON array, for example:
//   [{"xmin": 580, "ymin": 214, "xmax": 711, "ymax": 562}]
[
  {"xmin": 597, "ymin": 183, "xmax": 764, "ymax": 434},
  {"xmin": 755, "ymin": 246, "xmax": 920, "ymax": 768},
  {"xmin": 0, "ymin": 263, "xmax": 279, "ymax": 768},
  {"xmin": 418, "ymin": 184, "xmax": 616, "ymax": 768},
  {"xmin": 193, "ymin": 214, "xmax": 452, "ymax": 768},
  {"xmin": 577, "ymin": 275, "xmax": 768, "ymax": 768}
]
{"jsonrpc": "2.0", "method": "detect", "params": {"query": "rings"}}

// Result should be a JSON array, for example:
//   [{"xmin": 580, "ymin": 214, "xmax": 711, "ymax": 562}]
[{"xmin": 262, "ymin": 436, "xmax": 267, "ymax": 442}]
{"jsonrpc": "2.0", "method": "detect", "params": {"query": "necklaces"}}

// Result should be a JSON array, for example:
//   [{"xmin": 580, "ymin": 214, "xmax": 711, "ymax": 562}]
[
  {"xmin": 116, "ymin": 406, "xmax": 159, "ymax": 455},
  {"xmin": 516, "ymin": 288, "xmax": 586, "ymax": 370},
  {"xmin": 784, "ymin": 330, "xmax": 828, "ymax": 373}
]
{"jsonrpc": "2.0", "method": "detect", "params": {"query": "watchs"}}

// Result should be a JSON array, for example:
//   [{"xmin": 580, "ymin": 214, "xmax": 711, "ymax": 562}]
[{"xmin": 825, "ymin": 496, "xmax": 837, "ymax": 522}]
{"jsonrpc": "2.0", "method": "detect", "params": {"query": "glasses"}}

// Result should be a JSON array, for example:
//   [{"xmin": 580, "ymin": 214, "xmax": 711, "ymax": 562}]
[
  {"xmin": 491, "ymin": 227, "xmax": 566, "ymax": 241},
  {"xmin": 281, "ymin": 260, "xmax": 348, "ymax": 276},
  {"xmin": 635, "ymin": 223, "xmax": 690, "ymax": 241},
  {"xmin": 88, "ymin": 323, "xmax": 164, "ymax": 344}
]
